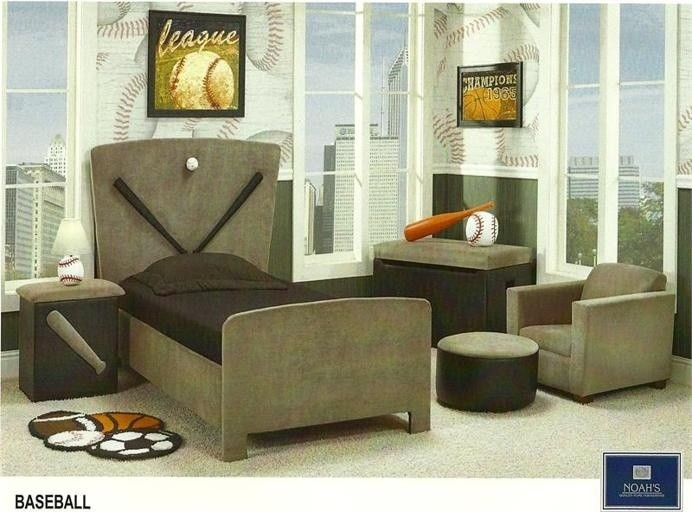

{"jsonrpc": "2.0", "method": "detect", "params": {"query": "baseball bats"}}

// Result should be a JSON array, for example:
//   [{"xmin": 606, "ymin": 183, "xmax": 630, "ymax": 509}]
[
  {"xmin": 113, "ymin": 177, "xmax": 188, "ymax": 254},
  {"xmin": 404, "ymin": 201, "xmax": 495, "ymax": 242},
  {"xmin": 194, "ymin": 172, "xmax": 263, "ymax": 253}
]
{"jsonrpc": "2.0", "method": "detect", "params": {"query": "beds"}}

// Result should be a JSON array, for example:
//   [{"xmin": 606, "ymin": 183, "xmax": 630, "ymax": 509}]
[{"xmin": 88, "ymin": 137, "xmax": 437, "ymax": 465}]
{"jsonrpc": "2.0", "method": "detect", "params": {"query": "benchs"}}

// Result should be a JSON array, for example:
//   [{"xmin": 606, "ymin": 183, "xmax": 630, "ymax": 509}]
[{"xmin": 367, "ymin": 235, "xmax": 537, "ymax": 351}]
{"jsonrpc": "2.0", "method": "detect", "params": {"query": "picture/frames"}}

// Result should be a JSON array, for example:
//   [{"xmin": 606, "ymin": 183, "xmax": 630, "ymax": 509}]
[
  {"xmin": 141, "ymin": 6, "xmax": 250, "ymax": 122},
  {"xmin": 455, "ymin": 60, "xmax": 524, "ymax": 129}
]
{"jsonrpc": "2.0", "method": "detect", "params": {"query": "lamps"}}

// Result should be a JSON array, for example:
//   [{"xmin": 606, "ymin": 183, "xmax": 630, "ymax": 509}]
[{"xmin": 50, "ymin": 220, "xmax": 94, "ymax": 289}]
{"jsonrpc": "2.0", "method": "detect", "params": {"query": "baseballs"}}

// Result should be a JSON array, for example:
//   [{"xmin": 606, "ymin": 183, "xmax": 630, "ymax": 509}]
[
  {"xmin": 46, "ymin": 311, "xmax": 106, "ymax": 374},
  {"xmin": 58, "ymin": 257, "xmax": 83, "ymax": 286},
  {"xmin": 44, "ymin": 430, "xmax": 105, "ymax": 451},
  {"xmin": 169, "ymin": 51, "xmax": 234, "ymax": 109},
  {"xmin": 187, "ymin": 158, "xmax": 198, "ymax": 171},
  {"xmin": 466, "ymin": 212, "xmax": 498, "ymax": 247}
]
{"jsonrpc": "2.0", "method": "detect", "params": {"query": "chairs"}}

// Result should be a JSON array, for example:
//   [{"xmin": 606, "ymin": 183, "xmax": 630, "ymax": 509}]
[{"xmin": 503, "ymin": 261, "xmax": 678, "ymax": 407}]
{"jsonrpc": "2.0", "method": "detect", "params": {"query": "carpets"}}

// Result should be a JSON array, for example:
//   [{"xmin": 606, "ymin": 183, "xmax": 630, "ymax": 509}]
[{"xmin": 24, "ymin": 405, "xmax": 188, "ymax": 461}]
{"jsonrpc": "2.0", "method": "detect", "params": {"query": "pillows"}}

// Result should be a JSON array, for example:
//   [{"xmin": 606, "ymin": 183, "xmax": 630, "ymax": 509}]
[{"xmin": 127, "ymin": 245, "xmax": 291, "ymax": 298}]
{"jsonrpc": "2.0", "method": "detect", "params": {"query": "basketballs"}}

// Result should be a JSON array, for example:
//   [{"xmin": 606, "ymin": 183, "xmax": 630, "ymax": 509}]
[
  {"xmin": 463, "ymin": 87, "xmax": 501, "ymax": 120},
  {"xmin": 89, "ymin": 412, "xmax": 163, "ymax": 434}
]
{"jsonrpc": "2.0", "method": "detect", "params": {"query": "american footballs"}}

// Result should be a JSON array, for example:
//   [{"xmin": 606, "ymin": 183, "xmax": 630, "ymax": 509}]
[{"xmin": 28, "ymin": 411, "xmax": 103, "ymax": 439}]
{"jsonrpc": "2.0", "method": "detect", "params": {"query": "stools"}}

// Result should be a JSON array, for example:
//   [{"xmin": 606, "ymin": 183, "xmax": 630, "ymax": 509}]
[
  {"xmin": 15, "ymin": 276, "xmax": 128, "ymax": 403},
  {"xmin": 432, "ymin": 328, "xmax": 542, "ymax": 414}
]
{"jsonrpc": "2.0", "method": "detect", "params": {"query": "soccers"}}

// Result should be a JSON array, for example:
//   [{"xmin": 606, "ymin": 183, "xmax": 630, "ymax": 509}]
[{"xmin": 86, "ymin": 430, "xmax": 181, "ymax": 460}]
